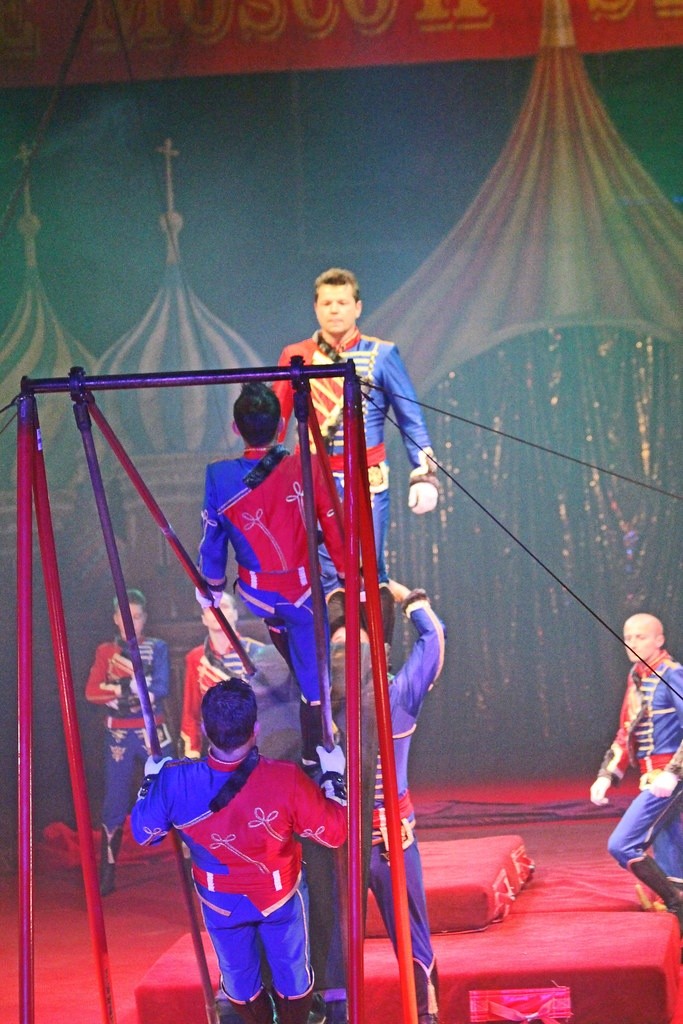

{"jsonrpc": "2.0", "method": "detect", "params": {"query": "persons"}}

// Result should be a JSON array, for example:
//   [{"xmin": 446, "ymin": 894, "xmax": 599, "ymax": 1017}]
[
  {"xmin": 295, "ymin": 581, "xmax": 446, "ymax": 1024},
  {"xmin": 589, "ymin": 613, "xmax": 682, "ymax": 964},
  {"xmin": 86, "ymin": 590, "xmax": 180, "ymax": 896},
  {"xmin": 265, "ymin": 267, "xmax": 440, "ymax": 673},
  {"xmin": 174, "ymin": 593, "xmax": 271, "ymax": 761},
  {"xmin": 191, "ymin": 379, "xmax": 363, "ymax": 786},
  {"xmin": 131, "ymin": 677, "xmax": 347, "ymax": 1024}
]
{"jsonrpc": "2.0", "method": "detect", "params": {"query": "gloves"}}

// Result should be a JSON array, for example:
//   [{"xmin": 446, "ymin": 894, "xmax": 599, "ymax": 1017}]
[
  {"xmin": 409, "ymin": 482, "xmax": 438, "ymax": 514},
  {"xmin": 129, "ymin": 690, "xmax": 154, "ymax": 713},
  {"xmin": 316, "ymin": 745, "xmax": 346, "ymax": 776},
  {"xmin": 144, "ymin": 755, "xmax": 173, "ymax": 777},
  {"xmin": 130, "ymin": 675, "xmax": 152, "ymax": 694},
  {"xmin": 589, "ymin": 777, "xmax": 612, "ymax": 806},
  {"xmin": 195, "ymin": 587, "xmax": 223, "ymax": 609},
  {"xmin": 649, "ymin": 772, "xmax": 678, "ymax": 797}
]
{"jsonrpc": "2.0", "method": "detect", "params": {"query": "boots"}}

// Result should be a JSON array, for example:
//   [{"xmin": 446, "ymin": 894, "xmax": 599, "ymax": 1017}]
[
  {"xmin": 627, "ymin": 853, "xmax": 683, "ymax": 921},
  {"xmin": 272, "ymin": 964, "xmax": 314, "ymax": 1023},
  {"xmin": 412, "ymin": 953, "xmax": 439, "ymax": 1024},
  {"xmin": 360, "ymin": 582, "xmax": 394, "ymax": 671},
  {"xmin": 299, "ymin": 695, "xmax": 323, "ymax": 778},
  {"xmin": 219, "ymin": 974, "xmax": 274, "ymax": 1024},
  {"xmin": 666, "ymin": 877, "xmax": 683, "ymax": 939},
  {"xmin": 325, "ymin": 586, "xmax": 347, "ymax": 637},
  {"xmin": 98, "ymin": 823, "xmax": 124, "ymax": 895},
  {"xmin": 264, "ymin": 620, "xmax": 294, "ymax": 678}
]
